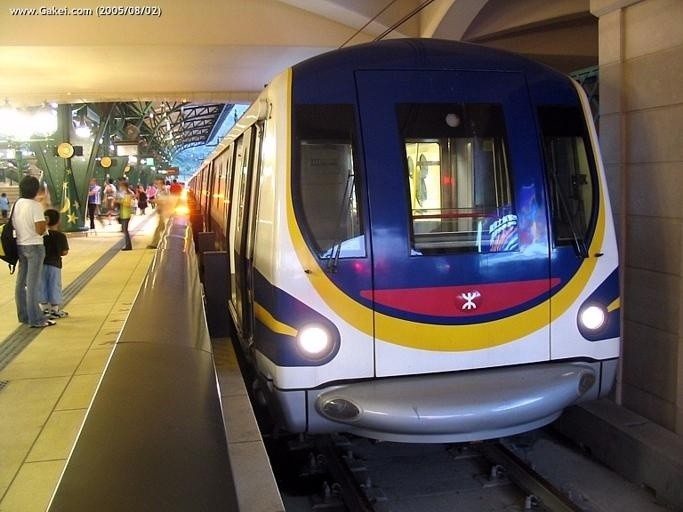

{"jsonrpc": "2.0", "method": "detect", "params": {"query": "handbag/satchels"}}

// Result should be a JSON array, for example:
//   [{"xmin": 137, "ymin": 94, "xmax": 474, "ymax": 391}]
[{"xmin": 0, "ymin": 217, "xmax": 19, "ymax": 266}]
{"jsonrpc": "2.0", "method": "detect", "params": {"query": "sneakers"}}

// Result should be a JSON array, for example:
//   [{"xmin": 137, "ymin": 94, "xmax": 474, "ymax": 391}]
[{"xmin": 30, "ymin": 309, "xmax": 68, "ymax": 327}]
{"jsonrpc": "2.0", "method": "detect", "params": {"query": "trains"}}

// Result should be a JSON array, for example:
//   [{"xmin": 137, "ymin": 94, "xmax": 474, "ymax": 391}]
[{"xmin": 182, "ymin": 35, "xmax": 625, "ymax": 445}]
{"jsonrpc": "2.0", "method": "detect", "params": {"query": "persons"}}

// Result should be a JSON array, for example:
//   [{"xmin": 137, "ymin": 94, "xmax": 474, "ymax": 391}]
[
  {"xmin": 8, "ymin": 175, "xmax": 57, "ymax": 328},
  {"xmin": 36, "ymin": 185, "xmax": 50, "ymax": 210},
  {"xmin": 84, "ymin": 177, "xmax": 185, "ymax": 231},
  {"xmin": 0, "ymin": 192, "xmax": 10, "ymax": 219},
  {"xmin": 113, "ymin": 180, "xmax": 133, "ymax": 251},
  {"xmin": 37, "ymin": 209, "xmax": 69, "ymax": 319},
  {"xmin": 145, "ymin": 178, "xmax": 172, "ymax": 249}
]
